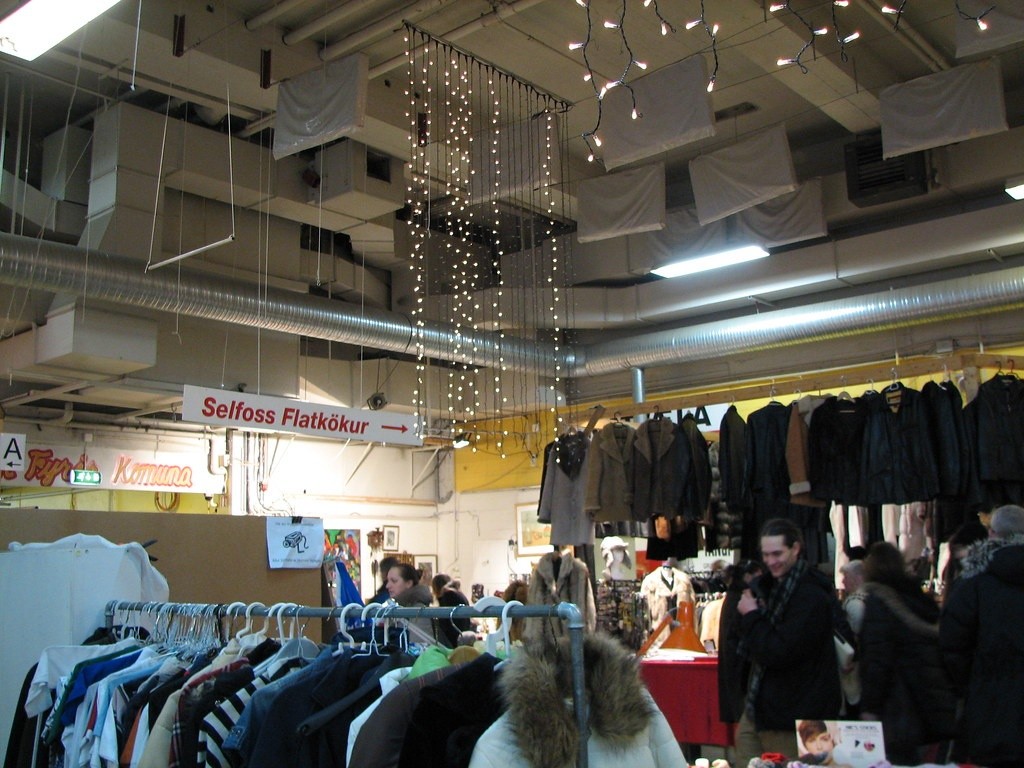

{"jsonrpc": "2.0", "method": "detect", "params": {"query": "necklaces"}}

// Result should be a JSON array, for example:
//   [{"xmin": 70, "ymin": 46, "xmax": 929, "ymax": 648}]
[{"xmin": 823, "ymin": 757, "xmax": 833, "ymax": 766}]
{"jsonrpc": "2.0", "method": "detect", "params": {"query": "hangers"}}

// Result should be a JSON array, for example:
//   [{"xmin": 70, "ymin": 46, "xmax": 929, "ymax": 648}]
[
  {"xmin": 42, "ymin": 591, "xmax": 591, "ymax": 738},
  {"xmin": 560, "ymin": 358, "xmax": 1024, "ymax": 438},
  {"xmin": 605, "ymin": 571, "xmax": 736, "ymax": 607}
]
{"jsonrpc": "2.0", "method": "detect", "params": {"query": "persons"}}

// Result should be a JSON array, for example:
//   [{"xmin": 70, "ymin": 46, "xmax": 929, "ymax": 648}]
[
  {"xmin": 641, "ymin": 560, "xmax": 695, "ymax": 634},
  {"xmin": 601, "ymin": 536, "xmax": 633, "ymax": 580},
  {"xmin": 699, "ymin": 505, "xmax": 1024, "ymax": 768},
  {"xmin": 365, "ymin": 556, "xmax": 471, "ymax": 645},
  {"xmin": 799, "ymin": 720, "xmax": 852, "ymax": 768}
]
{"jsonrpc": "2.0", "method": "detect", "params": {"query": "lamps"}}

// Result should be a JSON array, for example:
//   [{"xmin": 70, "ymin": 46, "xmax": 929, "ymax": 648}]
[
  {"xmin": 650, "ymin": 242, "xmax": 771, "ymax": 284},
  {"xmin": 1005, "ymin": 177, "xmax": 1024, "ymax": 200},
  {"xmin": 451, "ymin": 432, "xmax": 473, "ymax": 449},
  {"xmin": 237, "ymin": 382, "xmax": 248, "ymax": 391},
  {"xmin": 367, "ymin": 392, "xmax": 391, "ymax": 410}
]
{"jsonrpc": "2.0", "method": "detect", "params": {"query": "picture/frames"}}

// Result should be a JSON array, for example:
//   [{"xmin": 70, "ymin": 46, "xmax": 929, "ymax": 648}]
[
  {"xmin": 414, "ymin": 554, "xmax": 439, "ymax": 587},
  {"xmin": 517, "ymin": 500, "xmax": 564, "ymax": 557},
  {"xmin": 385, "ymin": 525, "xmax": 399, "ymax": 550}
]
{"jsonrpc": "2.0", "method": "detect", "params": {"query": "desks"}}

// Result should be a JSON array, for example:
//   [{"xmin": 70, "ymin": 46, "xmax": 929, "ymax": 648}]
[{"xmin": 639, "ymin": 651, "xmax": 740, "ymax": 748}]
